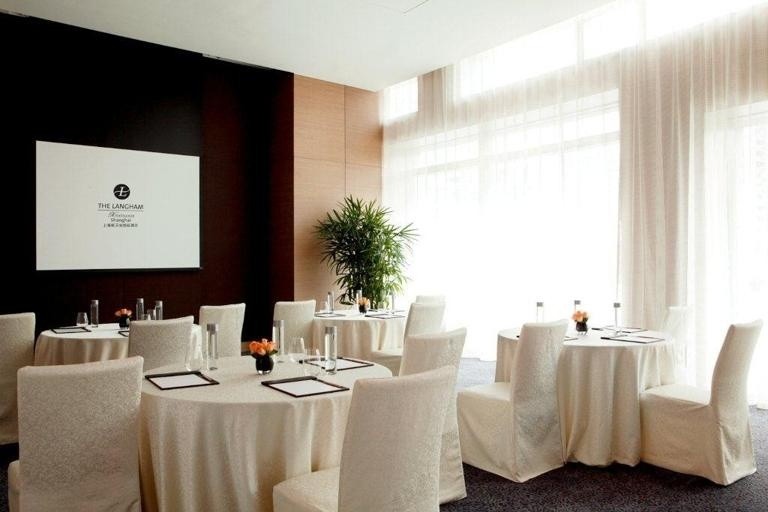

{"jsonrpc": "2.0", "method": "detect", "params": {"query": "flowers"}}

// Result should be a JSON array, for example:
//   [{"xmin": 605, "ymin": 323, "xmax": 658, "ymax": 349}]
[{"xmin": 571, "ymin": 311, "xmax": 590, "ymax": 323}]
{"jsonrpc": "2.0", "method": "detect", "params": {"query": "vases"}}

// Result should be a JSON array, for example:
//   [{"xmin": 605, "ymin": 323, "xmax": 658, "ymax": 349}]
[{"xmin": 576, "ymin": 322, "xmax": 586, "ymax": 332}]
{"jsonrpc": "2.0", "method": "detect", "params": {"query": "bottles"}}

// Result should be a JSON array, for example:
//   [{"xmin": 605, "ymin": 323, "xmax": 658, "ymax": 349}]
[
  {"xmin": 613, "ymin": 302, "xmax": 623, "ymax": 330},
  {"xmin": 573, "ymin": 300, "xmax": 581, "ymax": 323},
  {"xmin": 271, "ymin": 318, "xmax": 286, "ymax": 364},
  {"xmin": 356, "ymin": 288, "xmax": 363, "ymax": 306},
  {"xmin": 183, "ymin": 323, "xmax": 203, "ymax": 371},
  {"xmin": 89, "ymin": 297, "xmax": 100, "ymax": 328},
  {"xmin": 76, "ymin": 311, "xmax": 89, "ymax": 327},
  {"xmin": 205, "ymin": 321, "xmax": 219, "ymax": 372},
  {"xmin": 322, "ymin": 325, "xmax": 339, "ymax": 373},
  {"xmin": 135, "ymin": 297, "xmax": 164, "ymax": 322},
  {"xmin": 536, "ymin": 302, "xmax": 544, "ymax": 322},
  {"xmin": 324, "ymin": 290, "xmax": 333, "ymax": 313}
]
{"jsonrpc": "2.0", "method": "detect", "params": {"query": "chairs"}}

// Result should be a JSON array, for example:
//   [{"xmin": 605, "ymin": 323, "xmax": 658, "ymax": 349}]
[{"xmin": 638, "ymin": 319, "xmax": 763, "ymax": 485}]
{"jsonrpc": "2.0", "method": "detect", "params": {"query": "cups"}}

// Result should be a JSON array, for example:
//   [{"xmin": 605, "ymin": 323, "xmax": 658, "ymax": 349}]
[
  {"xmin": 286, "ymin": 338, "xmax": 306, "ymax": 360},
  {"xmin": 302, "ymin": 349, "xmax": 320, "ymax": 374}
]
